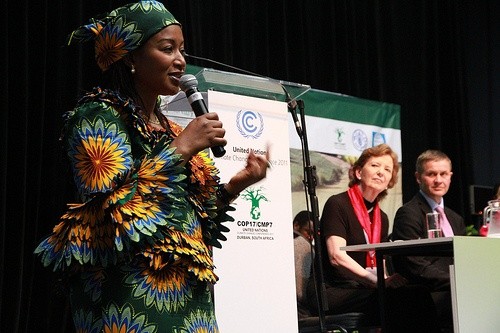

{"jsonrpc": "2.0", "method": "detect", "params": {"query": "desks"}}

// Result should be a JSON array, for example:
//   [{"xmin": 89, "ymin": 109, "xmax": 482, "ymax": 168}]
[{"xmin": 340, "ymin": 236, "xmax": 500, "ymax": 333}]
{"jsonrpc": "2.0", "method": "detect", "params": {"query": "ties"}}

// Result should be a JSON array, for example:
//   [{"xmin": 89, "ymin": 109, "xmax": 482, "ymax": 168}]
[{"xmin": 436, "ymin": 206, "xmax": 455, "ymax": 237}]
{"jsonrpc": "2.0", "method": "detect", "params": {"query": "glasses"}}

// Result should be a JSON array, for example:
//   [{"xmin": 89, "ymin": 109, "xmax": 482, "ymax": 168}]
[{"xmin": 303, "ymin": 227, "xmax": 314, "ymax": 236}]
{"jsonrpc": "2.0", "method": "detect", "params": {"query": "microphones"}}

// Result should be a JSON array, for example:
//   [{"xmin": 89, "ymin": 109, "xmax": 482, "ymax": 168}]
[{"xmin": 179, "ymin": 74, "xmax": 226, "ymax": 158}]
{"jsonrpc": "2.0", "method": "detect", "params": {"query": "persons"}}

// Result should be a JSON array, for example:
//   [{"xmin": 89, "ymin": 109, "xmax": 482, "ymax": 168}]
[
  {"xmin": 33, "ymin": 0, "xmax": 271, "ymax": 333},
  {"xmin": 306, "ymin": 145, "xmax": 420, "ymax": 333},
  {"xmin": 391, "ymin": 150, "xmax": 467, "ymax": 333},
  {"xmin": 293, "ymin": 210, "xmax": 320, "ymax": 310}
]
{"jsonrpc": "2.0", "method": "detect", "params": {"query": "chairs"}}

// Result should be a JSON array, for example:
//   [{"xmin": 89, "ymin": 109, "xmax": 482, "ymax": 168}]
[{"xmin": 293, "ymin": 229, "xmax": 363, "ymax": 333}]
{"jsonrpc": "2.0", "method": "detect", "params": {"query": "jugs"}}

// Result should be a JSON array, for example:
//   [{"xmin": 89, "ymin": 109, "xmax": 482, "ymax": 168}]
[{"xmin": 483, "ymin": 201, "xmax": 500, "ymax": 238}]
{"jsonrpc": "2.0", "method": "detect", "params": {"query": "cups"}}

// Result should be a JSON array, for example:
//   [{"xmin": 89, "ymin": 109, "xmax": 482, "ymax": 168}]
[{"xmin": 426, "ymin": 211, "xmax": 441, "ymax": 239}]
